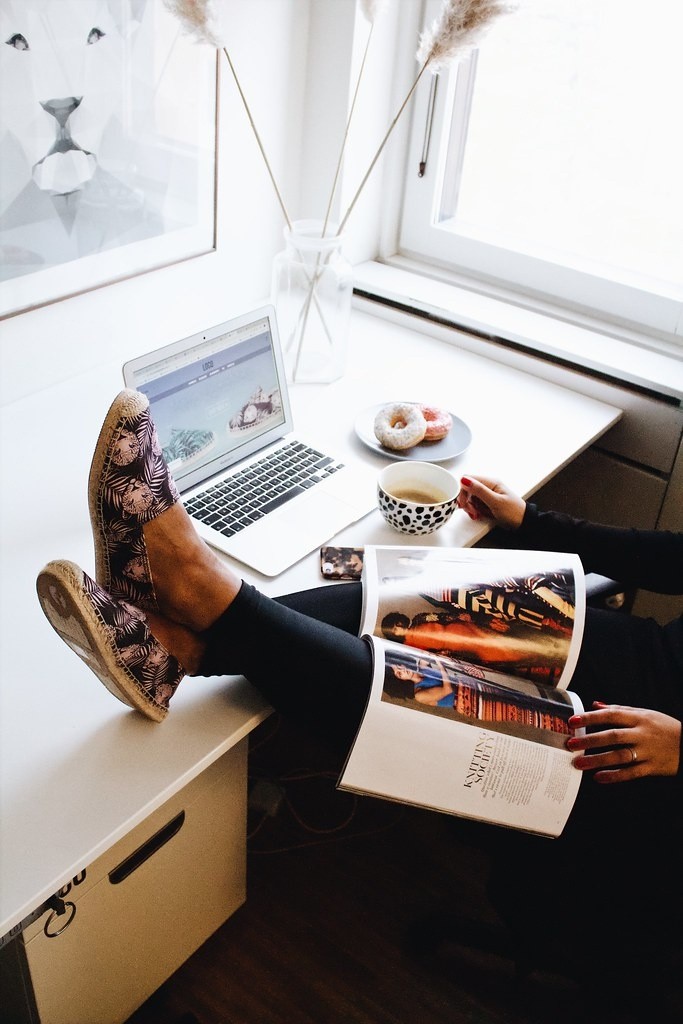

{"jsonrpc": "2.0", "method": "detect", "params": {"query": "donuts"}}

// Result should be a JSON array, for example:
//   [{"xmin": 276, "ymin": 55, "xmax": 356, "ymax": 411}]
[
  {"xmin": 374, "ymin": 402, "xmax": 427, "ymax": 450},
  {"xmin": 402, "ymin": 403, "xmax": 453, "ymax": 441}
]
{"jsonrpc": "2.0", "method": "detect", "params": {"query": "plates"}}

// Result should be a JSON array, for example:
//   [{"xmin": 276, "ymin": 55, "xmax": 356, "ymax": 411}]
[{"xmin": 356, "ymin": 401, "xmax": 474, "ymax": 462}]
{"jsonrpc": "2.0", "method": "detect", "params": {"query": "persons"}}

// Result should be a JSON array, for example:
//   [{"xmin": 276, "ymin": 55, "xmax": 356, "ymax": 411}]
[
  {"xmin": 380, "ymin": 569, "xmax": 575, "ymax": 687},
  {"xmin": 37, "ymin": 389, "xmax": 683, "ymax": 959},
  {"xmin": 382, "ymin": 652, "xmax": 575, "ymax": 735}
]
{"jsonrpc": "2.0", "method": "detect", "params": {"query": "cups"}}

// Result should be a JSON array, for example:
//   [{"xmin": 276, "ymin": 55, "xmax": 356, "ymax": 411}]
[{"xmin": 377, "ymin": 460, "xmax": 461, "ymax": 536}]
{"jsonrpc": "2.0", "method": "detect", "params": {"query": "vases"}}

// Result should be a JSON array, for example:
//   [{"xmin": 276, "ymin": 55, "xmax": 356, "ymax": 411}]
[{"xmin": 266, "ymin": 219, "xmax": 357, "ymax": 389}]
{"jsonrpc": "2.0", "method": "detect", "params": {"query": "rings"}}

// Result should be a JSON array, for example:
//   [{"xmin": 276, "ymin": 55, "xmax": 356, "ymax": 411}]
[{"xmin": 629, "ymin": 746, "xmax": 637, "ymax": 763}]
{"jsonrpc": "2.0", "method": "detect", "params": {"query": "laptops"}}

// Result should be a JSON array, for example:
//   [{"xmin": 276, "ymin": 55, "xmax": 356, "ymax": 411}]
[{"xmin": 120, "ymin": 305, "xmax": 383, "ymax": 576}]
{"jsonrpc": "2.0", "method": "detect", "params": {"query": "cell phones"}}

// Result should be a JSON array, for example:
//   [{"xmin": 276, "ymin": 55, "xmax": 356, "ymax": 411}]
[{"xmin": 318, "ymin": 545, "xmax": 365, "ymax": 581}]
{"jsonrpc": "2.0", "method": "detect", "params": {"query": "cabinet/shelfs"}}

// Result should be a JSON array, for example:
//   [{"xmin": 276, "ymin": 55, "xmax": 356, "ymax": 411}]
[{"xmin": 0, "ymin": 295, "xmax": 630, "ymax": 1024}]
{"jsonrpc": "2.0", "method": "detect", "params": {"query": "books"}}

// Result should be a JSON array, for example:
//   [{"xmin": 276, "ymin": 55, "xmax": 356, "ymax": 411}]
[{"xmin": 336, "ymin": 546, "xmax": 586, "ymax": 839}]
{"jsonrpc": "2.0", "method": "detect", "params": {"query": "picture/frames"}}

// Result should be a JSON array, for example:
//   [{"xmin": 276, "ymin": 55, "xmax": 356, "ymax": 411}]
[{"xmin": 0, "ymin": 0, "xmax": 220, "ymax": 319}]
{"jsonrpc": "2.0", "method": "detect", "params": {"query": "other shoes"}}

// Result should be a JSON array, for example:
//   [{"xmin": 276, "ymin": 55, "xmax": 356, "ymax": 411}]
[
  {"xmin": 36, "ymin": 559, "xmax": 186, "ymax": 723},
  {"xmin": 87, "ymin": 388, "xmax": 180, "ymax": 615}
]
